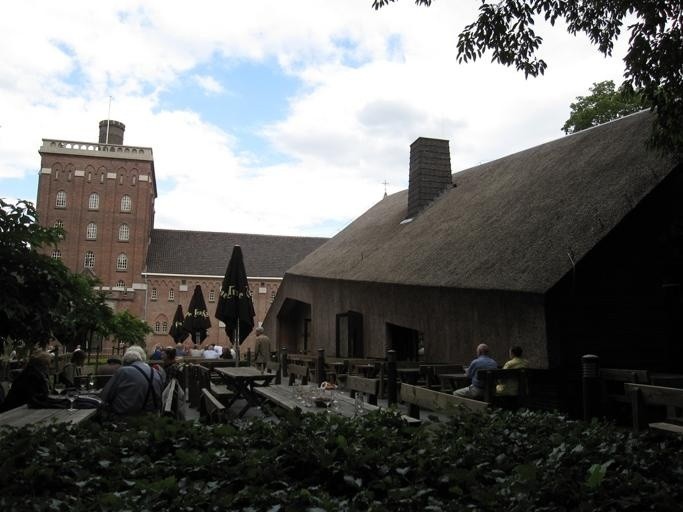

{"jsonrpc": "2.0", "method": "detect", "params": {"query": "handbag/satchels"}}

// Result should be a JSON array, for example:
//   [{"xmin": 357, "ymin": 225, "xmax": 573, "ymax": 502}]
[
  {"xmin": 28, "ymin": 394, "xmax": 71, "ymax": 409},
  {"xmin": 72, "ymin": 398, "xmax": 102, "ymax": 409}
]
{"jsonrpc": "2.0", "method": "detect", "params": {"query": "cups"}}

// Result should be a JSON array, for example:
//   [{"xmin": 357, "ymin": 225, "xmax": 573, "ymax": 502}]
[
  {"xmin": 290, "ymin": 379, "xmax": 343, "ymax": 413},
  {"xmin": 354, "ymin": 391, "xmax": 365, "ymax": 414}
]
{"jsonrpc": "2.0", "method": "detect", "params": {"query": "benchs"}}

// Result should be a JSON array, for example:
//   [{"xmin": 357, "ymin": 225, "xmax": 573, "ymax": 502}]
[
  {"xmin": 255, "ymin": 347, "xmax": 533, "ymax": 426},
  {"xmin": 579, "ymin": 353, "xmax": 682, "ymax": 442},
  {"xmin": 0, "ymin": 345, "xmax": 103, "ymax": 432},
  {"xmin": 147, "ymin": 348, "xmax": 281, "ymax": 427}
]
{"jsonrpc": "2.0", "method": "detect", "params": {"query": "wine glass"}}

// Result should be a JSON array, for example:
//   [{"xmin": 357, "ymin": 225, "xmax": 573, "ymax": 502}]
[
  {"xmin": 66, "ymin": 387, "xmax": 80, "ymax": 411},
  {"xmin": 54, "ymin": 383, "xmax": 65, "ymax": 399},
  {"xmin": 80, "ymin": 374, "xmax": 97, "ymax": 394}
]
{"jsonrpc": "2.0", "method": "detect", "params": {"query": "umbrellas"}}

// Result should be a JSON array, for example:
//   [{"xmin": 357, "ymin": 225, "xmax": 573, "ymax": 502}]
[
  {"xmin": 191, "ymin": 328, "xmax": 207, "ymax": 346},
  {"xmin": 168, "ymin": 304, "xmax": 188, "ymax": 343},
  {"xmin": 225, "ymin": 320, "xmax": 254, "ymax": 346},
  {"xmin": 182, "ymin": 284, "xmax": 211, "ymax": 332},
  {"xmin": 213, "ymin": 244, "xmax": 254, "ymax": 328}
]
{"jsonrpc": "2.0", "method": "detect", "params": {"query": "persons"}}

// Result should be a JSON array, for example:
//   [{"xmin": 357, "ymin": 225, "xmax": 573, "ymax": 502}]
[
  {"xmin": 3, "ymin": 350, "xmax": 50, "ymax": 409},
  {"xmin": 495, "ymin": 345, "xmax": 529, "ymax": 397},
  {"xmin": 454, "ymin": 343, "xmax": 496, "ymax": 399},
  {"xmin": 253, "ymin": 327, "xmax": 271, "ymax": 369},
  {"xmin": 63, "ymin": 343, "xmax": 235, "ymax": 426}
]
